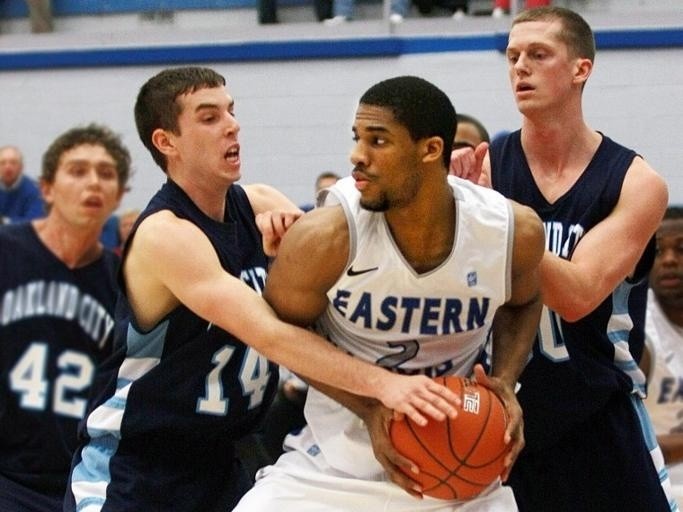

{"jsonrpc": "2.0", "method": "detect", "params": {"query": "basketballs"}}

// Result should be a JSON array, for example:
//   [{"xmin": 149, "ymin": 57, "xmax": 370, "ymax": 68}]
[{"xmin": 390, "ymin": 376, "xmax": 517, "ymax": 502}]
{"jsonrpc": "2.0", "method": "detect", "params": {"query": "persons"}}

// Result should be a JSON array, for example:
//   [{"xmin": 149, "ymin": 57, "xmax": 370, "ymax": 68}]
[
  {"xmin": 451, "ymin": 6, "xmax": 679, "ymax": 512},
  {"xmin": 637, "ymin": 204, "xmax": 682, "ymax": 501},
  {"xmin": 113, "ymin": 209, "xmax": 140, "ymax": 254},
  {"xmin": 0, "ymin": 147, "xmax": 45, "ymax": 225},
  {"xmin": 452, "ymin": 113, "xmax": 490, "ymax": 151},
  {"xmin": 0, "ymin": 123, "xmax": 130, "ymax": 512},
  {"xmin": 315, "ymin": 171, "xmax": 340, "ymax": 194},
  {"xmin": 64, "ymin": 66, "xmax": 462, "ymax": 512},
  {"xmin": 232, "ymin": 76, "xmax": 547, "ymax": 512}
]
